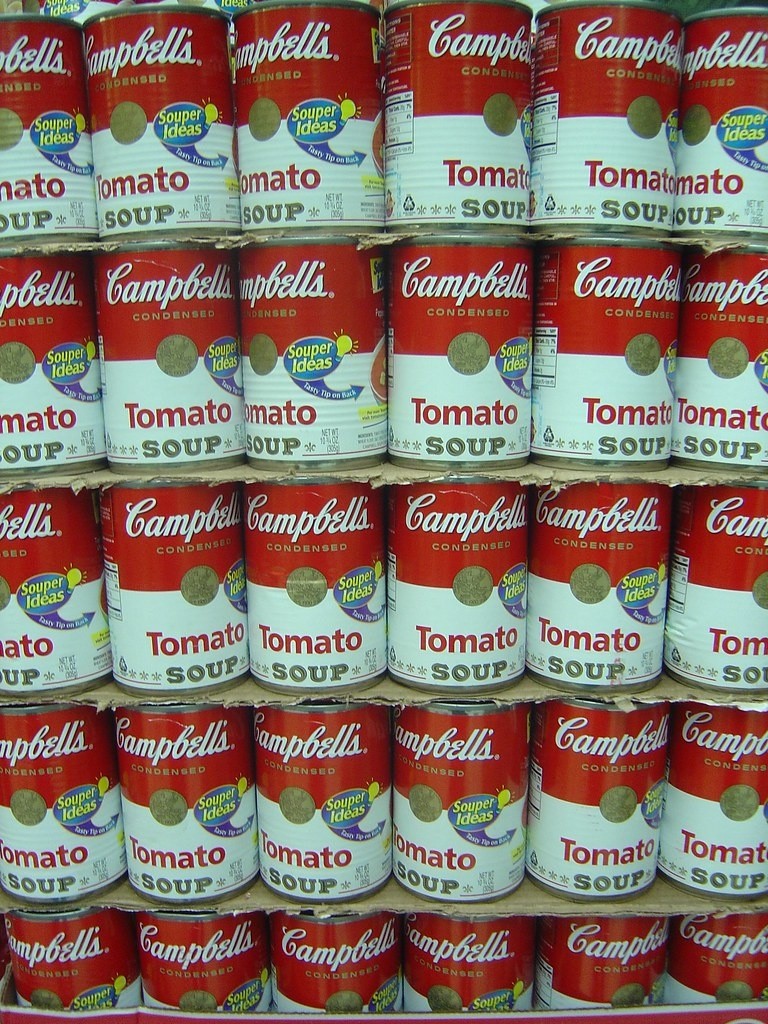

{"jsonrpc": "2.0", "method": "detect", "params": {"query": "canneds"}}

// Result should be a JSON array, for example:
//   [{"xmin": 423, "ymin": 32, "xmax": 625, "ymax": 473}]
[{"xmin": 0, "ymin": 0, "xmax": 768, "ymax": 1024}]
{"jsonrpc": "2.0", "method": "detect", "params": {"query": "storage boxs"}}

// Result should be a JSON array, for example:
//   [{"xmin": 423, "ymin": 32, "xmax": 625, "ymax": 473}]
[{"xmin": 0, "ymin": 960, "xmax": 768, "ymax": 1024}]
